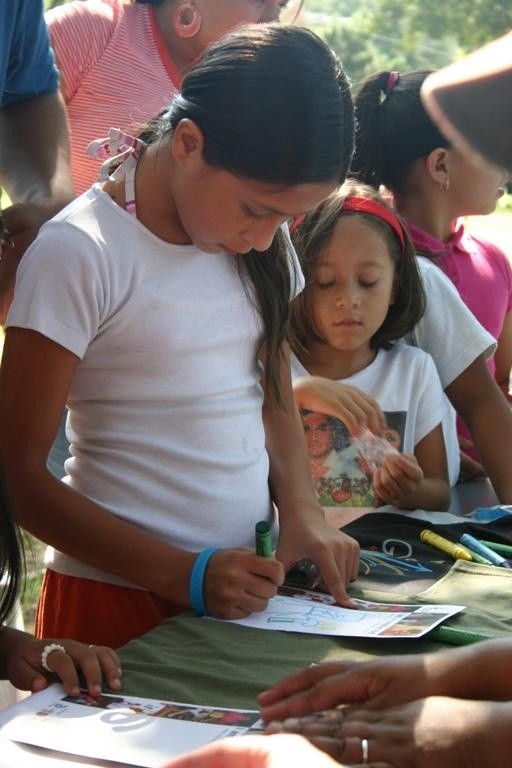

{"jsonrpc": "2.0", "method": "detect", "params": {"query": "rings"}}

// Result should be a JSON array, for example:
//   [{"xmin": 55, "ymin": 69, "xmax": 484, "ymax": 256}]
[
  {"xmin": 361, "ymin": 738, "xmax": 369, "ymax": 762},
  {"xmin": 89, "ymin": 644, "xmax": 95, "ymax": 648},
  {"xmin": 41, "ymin": 643, "xmax": 65, "ymax": 673}
]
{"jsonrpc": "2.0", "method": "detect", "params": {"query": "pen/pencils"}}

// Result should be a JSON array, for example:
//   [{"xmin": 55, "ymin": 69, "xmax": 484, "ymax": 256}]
[
  {"xmin": 420, "ymin": 529, "xmax": 512, "ymax": 569},
  {"xmin": 255, "ymin": 521, "xmax": 272, "ymax": 557},
  {"xmin": 422, "ymin": 625, "xmax": 492, "ymax": 646}
]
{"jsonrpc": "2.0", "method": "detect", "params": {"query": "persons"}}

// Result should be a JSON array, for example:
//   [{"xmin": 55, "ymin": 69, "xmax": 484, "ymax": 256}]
[
  {"xmin": 0, "ymin": 23, "xmax": 361, "ymax": 651},
  {"xmin": 333, "ymin": 70, "xmax": 511, "ymax": 512},
  {"xmin": 0, "ymin": 0, "xmax": 76, "ymax": 271},
  {"xmin": 41, "ymin": 1, "xmax": 295, "ymax": 212},
  {"xmin": 266, "ymin": 176, "xmax": 464, "ymax": 512},
  {"xmin": 253, "ymin": 628, "xmax": 511, "ymax": 768},
  {"xmin": 0, "ymin": 498, "xmax": 124, "ymax": 703}
]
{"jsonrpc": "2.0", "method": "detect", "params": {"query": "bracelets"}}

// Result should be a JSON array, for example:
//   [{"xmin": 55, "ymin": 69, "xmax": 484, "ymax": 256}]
[{"xmin": 188, "ymin": 544, "xmax": 224, "ymax": 619}]
